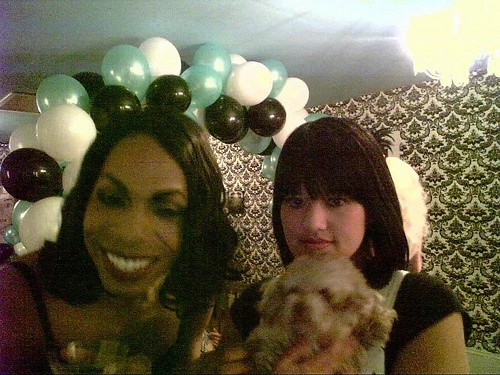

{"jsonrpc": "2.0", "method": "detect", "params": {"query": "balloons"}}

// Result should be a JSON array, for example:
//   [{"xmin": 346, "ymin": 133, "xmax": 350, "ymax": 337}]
[{"xmin": 2, "ymin": 37, "xmax": 311, "ymax": 258}]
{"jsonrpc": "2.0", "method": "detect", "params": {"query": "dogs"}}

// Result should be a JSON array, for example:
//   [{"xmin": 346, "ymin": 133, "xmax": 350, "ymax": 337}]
[{"xmin": 240, "ymin": 251, "xmax": 399, "ymax": 375}]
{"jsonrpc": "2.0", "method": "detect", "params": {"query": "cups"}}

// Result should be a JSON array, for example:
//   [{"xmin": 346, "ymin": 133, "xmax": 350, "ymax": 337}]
[{"xmin": 57, "ymin": 338, "xmax": 93, "ymax": 374}]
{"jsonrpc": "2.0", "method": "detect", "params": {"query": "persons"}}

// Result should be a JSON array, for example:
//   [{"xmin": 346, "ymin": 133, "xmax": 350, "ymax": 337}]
[
  {"xmin": 230, "ymin": 116, "xmax": 473, "ymax": 374},
  {"xmin": 0, "ymin": 105, "xmax": 249, "ymax": 375}
]
